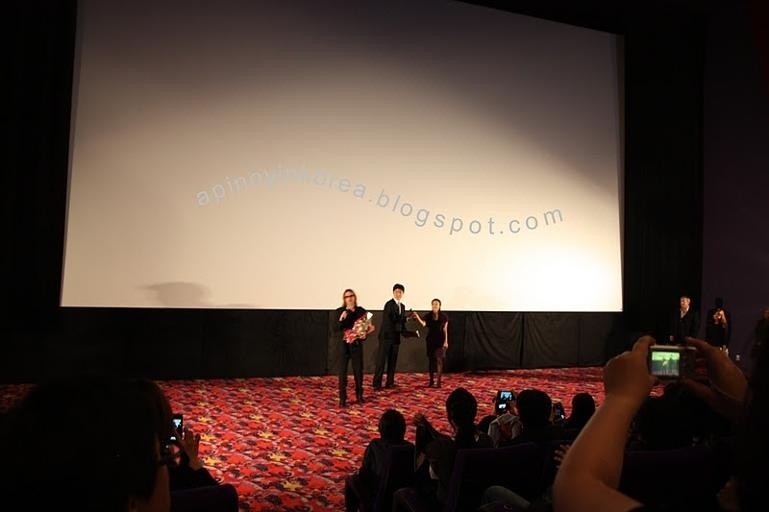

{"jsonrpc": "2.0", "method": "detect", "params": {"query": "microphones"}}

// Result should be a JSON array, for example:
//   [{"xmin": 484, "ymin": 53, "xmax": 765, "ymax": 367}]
[{"xmin": 410, "ymin": 307, "xmax": 417, "ymax": 321}]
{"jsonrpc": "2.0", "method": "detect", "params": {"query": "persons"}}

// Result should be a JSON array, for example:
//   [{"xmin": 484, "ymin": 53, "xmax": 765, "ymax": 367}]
[
  {"xmin": 334, "ymin": 289, "xmax": 375, "ymax": 409},
  {"xmin": 411, "ymin": 298, "xmax": 449, "ymax": 389},
  {"xmin": 668, "ymin": 296, "xmax": 700, "ymax": 348},
  {"xmin": 0, "ymin": 364, "xmax": 243, "ymax": 511},
  {"xmin": 705, "ymin": 296, "xmax": 733, "ymax": 347},
  {"xmin": 551, "ymin": 309, "xmax": 768, "ymax": 512},
  {"xmin": 372, "ymin": 283, "xmax": 421, "ymax": 389},
  {"xmin": 342, "ymin": 387, "xmax": 598, "ymax": 512}
]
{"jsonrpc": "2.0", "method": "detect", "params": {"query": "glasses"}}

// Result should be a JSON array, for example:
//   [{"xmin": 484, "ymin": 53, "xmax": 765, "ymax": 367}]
[
  {"xmin": 345, "ymin": 295, "xmax": 353, "ymax": 299},
  {"xmin": 157, "ymin": 444, "xmax": 185, "ymax": 470}
]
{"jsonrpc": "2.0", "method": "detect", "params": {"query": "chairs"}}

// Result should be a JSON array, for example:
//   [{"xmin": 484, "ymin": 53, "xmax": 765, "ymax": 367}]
[
  {"xmin": 175, "ymin": 485, "xmax": 239, "ymax": 512},
  {"xmin": 346, "ymin": 441, "xmax": 713, "ymax": 512}
]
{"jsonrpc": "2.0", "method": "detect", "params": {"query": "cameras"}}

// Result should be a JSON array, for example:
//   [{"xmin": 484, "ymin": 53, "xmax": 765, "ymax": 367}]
[
  {"xmin": 166, "ymin": 412, "xmax": 184, "ymax": 443},
  {"xmin": 496, "ymin": 390, "xmax": 513, "ymax": 413},
  {"xmin": 648, "ymin": 343, "xmax": 698, "ymax": 380}
]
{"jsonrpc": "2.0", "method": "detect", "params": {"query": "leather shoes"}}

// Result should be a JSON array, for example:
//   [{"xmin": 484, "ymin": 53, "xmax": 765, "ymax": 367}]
[
  {"xmin": 429, "ymin": 380, "xmax": 441, "ymax": 387},
  {"xmin": 374, "ymin": 384, "xmax": 396, "ymax": 391},
  {"xmin": 340, "ymin": 394, "xmax": 364, "ymax": 407}
]
